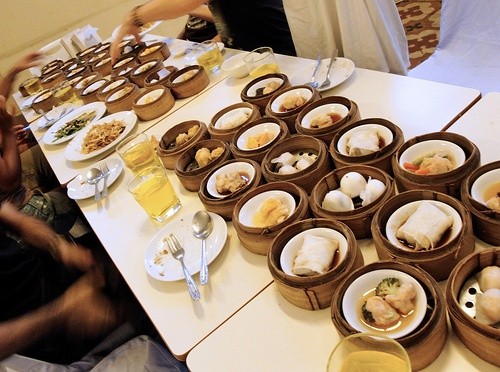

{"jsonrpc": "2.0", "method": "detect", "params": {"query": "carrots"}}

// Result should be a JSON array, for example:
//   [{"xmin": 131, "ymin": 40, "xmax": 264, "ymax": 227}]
[{"xmin": 404, "ymin": 162, "xmax": 431, "ymax": 175}]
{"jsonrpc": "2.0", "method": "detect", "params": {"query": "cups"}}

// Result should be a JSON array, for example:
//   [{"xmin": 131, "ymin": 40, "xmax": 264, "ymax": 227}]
[
  {"xmin": 326, "ymin": 333, "xmax": 411, "ymax": 372},
  {"xmin": 193, "ymin": 40, "xmax": 225, "ymax": 79},
  {"xmin": 13, "ymin": 68, "xmax": 44, "ymax": 99},
  {"xmin": 115, "ymin": 132, "xmax": 166, "ymax": 180},
  {"xmin": 242, "ymin": 47, "xmax": 281, "ymax": 79},
  {"xmin": 49, "ymin": 80, "xmax": 81, "ymax": 109},
  {"xmin": 126, "ymin": 166, "xmax": 183, "ymax": 226}
]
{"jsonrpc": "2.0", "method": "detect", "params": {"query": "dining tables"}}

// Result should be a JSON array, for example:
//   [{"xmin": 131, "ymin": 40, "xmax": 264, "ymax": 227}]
[{"xmin": 11, "ymin": 34, "xmax": 500, "ymax": 372}]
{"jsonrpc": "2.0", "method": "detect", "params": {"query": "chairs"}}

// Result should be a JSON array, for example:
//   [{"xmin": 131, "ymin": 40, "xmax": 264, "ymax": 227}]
[{"xmin": 407, "ymin": 0, "xmax": 500, "ymax": 93}]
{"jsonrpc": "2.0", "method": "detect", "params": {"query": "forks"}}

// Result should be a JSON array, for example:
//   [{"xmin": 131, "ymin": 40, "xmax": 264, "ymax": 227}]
[
  {"xmin": 164, "ymin": 233, "xmax": 201, "ymax": 302},
  {"xmin": 99, "ymin": 163, "xmax": 111, "ymax": 198},
  {"xmin": 318, "ymin": 49, "xmax": 339, "ymax": 89}
]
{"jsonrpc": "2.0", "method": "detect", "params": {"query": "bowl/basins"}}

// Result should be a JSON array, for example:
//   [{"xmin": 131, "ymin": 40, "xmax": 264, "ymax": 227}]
[
  {"xmin": 40, "ymin": 44, "xmax": 199, "ymax": 106},
  {"xmin": 221, "ymin": 52, "xmax": 248, "ymax": 79},
  {"xmin": 208, "ymin": 77, "xmax": 500, "ymax": 342}
]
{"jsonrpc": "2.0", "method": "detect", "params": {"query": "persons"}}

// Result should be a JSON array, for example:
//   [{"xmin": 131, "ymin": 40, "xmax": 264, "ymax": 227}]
[
  {"xmin": 0, "ymin": 51, "xmax": 190, "ymax": 372},
  {"xmin": 109, "ymin": 0, "xmax": 298, "ymax": 66}
]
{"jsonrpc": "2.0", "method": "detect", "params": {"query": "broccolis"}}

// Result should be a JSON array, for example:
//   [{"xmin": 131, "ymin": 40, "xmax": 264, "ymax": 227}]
[
  {"xmin": 375, "ymin": 277, "xmax": 400, "ymax": 298},
  {"xmin": 362, "ymin": 300, "xmax": 374, "ymax": 323}
]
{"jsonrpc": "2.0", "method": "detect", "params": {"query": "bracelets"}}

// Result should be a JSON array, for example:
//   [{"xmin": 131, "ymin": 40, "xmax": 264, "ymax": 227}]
[{"xmin": 129, "ymin": 6, "xmax": 143, "ymax": 27}]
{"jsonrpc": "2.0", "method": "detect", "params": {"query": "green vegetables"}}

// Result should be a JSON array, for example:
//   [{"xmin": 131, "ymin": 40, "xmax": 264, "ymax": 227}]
[{"xmin": 53, "ymin": 118, "xmax": 88, "ymax": 142}]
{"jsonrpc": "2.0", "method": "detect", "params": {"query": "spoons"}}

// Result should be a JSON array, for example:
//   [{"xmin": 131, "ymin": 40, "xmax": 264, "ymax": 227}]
[
  {"xmin": 86, "ymin": 167, "xmax": 103, "ymax": 201},
  {"xmin": 304, "ymin": 55, "xmax": 322, "ymax": 89},
  {"xmin": 191, "ymin": 211, "xmax": 214, "ymax": 286}
]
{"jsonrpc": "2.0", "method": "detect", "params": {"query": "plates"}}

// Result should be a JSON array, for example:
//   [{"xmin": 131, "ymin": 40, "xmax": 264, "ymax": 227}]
[
  {"xmin": 64, "ymin": 110, "xmax": 138, "ymax": 162},
  {"xmin": 111, "ymin": 20, "xmax": 164, "ymax": 40},
  {"xmin": 20, "ymin": 95, "xmax": 36, "ymax": 110},
  {"xmin": 292, "ymin": 58, "xmax": 354, "ymax": 91},
  {"xmin": 66, "ymin": 157, "xmax": 124, "ymax": 200},
  {"xmin": 185, "ymin": 41, "xmax": 224, "ymax": 66},
  {"xmin": 144, "ymin": 211, "xmax": 228, "ymax": 281},
  {"xmin": 42, "ymin": 101, "xmax": 107, "ymax": 145},
  {"xmin": 36, "ymin": 103, "xmax": 73, "ymax": 128}
]
